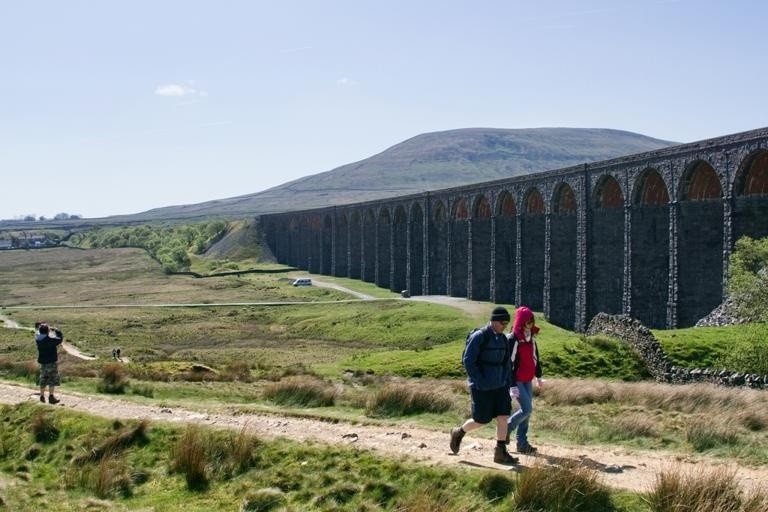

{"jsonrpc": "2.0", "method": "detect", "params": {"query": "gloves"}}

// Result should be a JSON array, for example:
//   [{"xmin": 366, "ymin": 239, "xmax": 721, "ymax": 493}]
[
  {"xmin": 510, "ymin": 386, "xmax": 520, "ymax": 399},
  {"xmin": 536, "ymin": 379, "xmax": 544, "ymax": 388}
]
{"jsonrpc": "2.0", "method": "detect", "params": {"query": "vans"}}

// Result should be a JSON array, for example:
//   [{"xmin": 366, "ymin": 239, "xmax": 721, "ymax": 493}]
[{"xmin": 293, "ymin": 278, "xmax": 312, "ymax": 286}]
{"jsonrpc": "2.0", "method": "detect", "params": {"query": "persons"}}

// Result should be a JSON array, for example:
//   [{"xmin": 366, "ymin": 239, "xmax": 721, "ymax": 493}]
[
  {"xmin": 449, "ymin": 307, "xmax": 519, "ymax": 466},
  {"xmin": 34, "ymin": 323, "xmax": 63, "ymax": 404},
  {"xmin": 116, "ymin": 347, "xmax": 120, "ymax": 358},
  {"xmin": 504, "ymin": 306, "xmax": 543, "ymax": 454},
  {"xmin": 111, "ymin": 347, "xmax": 116, "ymax": 357}
]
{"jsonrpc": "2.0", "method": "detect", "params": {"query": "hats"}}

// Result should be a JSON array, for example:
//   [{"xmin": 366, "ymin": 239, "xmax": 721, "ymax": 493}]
[{"xmin": 491, "ymin": 308, "xmax": 510, "ymax": 321}]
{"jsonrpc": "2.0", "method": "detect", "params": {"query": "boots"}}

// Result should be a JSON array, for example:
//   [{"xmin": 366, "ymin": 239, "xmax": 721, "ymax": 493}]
[
  {"xmin": 450, "ymin": 427, "xmax": 464, "ymax": 453},
  {"xmin": 494, "ymin": 444, "xmax": 517, "ymax": 465}
]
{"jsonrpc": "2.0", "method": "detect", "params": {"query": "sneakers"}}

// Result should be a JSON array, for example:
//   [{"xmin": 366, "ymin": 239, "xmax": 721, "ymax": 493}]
[
  {"xmin": 40, "ymin": 394, "xmax": 60, "ymax": 404},
  {"xmin": 506, "ymin": 429, "xmax": 509, "ymax": 444},
  {"xmin": 517, "ymin": 444, "xmax": 537, "ymax": 453}
]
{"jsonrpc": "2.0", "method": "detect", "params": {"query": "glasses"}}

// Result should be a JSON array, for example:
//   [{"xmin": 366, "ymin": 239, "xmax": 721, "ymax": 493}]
[{"xmin": 501, "ymin": 320, "xmax": 509, "ymax": 325}]
